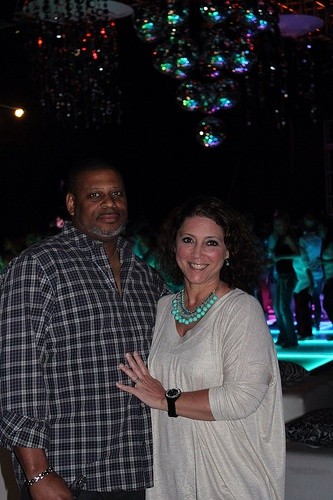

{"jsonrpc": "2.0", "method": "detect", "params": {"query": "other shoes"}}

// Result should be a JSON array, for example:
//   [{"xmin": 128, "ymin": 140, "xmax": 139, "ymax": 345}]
[
  {"xmin": 268, "ymin": 323, "xmax": 279, "ymax": 330},
  {"xmin": 282, "ymin": 342, "xmax": 299, "ymax": 350}
]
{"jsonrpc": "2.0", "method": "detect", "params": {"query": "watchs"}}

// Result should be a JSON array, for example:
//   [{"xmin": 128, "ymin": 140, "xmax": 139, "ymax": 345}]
[{"xmin": 165, "ymin": 388, "xmax": 181, "ymax": 417}]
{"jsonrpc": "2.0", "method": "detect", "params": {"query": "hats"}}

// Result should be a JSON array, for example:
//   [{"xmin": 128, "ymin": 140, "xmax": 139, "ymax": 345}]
[{"xmin": 301, "ymin": 220, "xmax": 313, "ymax": 230}]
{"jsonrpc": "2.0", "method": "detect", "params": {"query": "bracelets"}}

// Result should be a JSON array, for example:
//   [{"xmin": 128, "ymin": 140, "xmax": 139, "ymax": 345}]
[{"xmin": 23, "ymin": 466, "xmax": 54, "ymax": 488}]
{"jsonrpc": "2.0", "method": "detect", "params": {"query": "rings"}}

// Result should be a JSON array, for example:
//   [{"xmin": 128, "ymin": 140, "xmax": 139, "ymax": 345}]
[{"xmin": 134, "ymin": 379, "xmax": 139, "ymax": 383}]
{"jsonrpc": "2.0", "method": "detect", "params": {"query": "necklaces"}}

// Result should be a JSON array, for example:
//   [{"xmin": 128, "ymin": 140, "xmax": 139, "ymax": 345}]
[{"xmin": 171, "ymin": 280, "xmax": 223, "ymax": 325}]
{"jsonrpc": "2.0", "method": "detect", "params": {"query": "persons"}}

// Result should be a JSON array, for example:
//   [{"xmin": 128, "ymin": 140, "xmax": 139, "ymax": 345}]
[
  {"xmin": 4, "ymin": 213, "xmax": 333, "ymax": 348},
  {"xmin": 114, "ymin": 192, "xmax": 287, "ymax": 500},
  {"xmin": 0, "ymin": 158, "xmax": 172, "ymax": 500}
]
